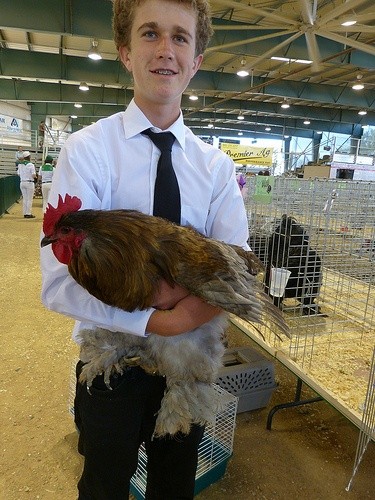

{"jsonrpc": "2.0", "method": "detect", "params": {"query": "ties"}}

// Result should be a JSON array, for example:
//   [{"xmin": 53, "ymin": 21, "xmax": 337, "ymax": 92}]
[{"xmin": 140, "ymin": 128, "xmax": 181, "ymax": 225}]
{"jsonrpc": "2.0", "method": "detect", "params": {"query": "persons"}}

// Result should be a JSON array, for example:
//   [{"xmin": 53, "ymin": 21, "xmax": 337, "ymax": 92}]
[
  {"xmin": 37, "ymin": 0, "xmax": 250, "ymax": 500},
  {"xmin": 15, "ymin": 145, "xmax": 37, "ymax": 219},
  {"xmin": 37, "ymin": 155, "xmax": 57, "ymax": 214},
  {"xmin": 38, "ymin": 120, "xmax": 44, "ymax": 148}
]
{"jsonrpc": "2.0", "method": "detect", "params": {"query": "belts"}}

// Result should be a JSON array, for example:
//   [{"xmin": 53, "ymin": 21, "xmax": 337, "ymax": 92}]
[
  {"xmin": 26, "ymin": 180, "xmax": 35, "ymax": 182},
  {"xmin": 42, "ymin": 181, "xmax": 52, "ymax": 183}
]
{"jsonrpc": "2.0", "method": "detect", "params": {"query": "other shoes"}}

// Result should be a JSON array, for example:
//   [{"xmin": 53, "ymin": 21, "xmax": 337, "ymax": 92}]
[{"xmin": 24, "ymin": 214, "xmax": 36, "ymax": 218}]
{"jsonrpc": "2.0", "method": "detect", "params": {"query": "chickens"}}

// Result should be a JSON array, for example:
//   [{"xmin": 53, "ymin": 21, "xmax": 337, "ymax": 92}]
[
  {"xmin": 39, "ymin": 191, "xmax": 293, "ymax": 444},
  {"xmin": 259, "ymin": 212, "xmax": 331, "ymax": 319}
]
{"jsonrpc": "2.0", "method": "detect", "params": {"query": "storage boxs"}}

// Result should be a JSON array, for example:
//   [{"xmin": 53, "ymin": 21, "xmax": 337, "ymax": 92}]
[{"xmin": 215, "ymin": 344, "xmax": 280, "ymax": 414}]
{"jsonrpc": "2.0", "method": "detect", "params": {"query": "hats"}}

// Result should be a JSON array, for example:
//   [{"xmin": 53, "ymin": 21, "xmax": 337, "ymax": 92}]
[
  {"xmin": 22, "ymin": 150, "xmax": 30, "ymax": 157},
  {"xmin": 45, "ymin": 155, "xmax": 53, "ymax": 161}
]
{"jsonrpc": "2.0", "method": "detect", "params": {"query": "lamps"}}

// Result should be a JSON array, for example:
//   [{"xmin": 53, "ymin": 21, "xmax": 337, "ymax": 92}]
[
  {"xmin": 280, "ymin": 96, "xmax": 291, "ymax": 108},
  {"xmin": 351, "ymin": 67, "xmax": 365, "ymax": 90},
  {"xmin": 189, "ymin": 88, "xmax": 199, "ymax": 100},
  {"xmin": 86, "ymin": 39, "xmax": 104, "ymax": 62},
  {"xmin": 237, "ymin": 100, "xmax": 244, "ymax": 120},
  {"xmin": 208, "ymin": 118, "xmax": 214, "ymax": 127},
  {"xmin": 237, "ymin": 56, "xmax": 250, "ymax": 77},
  {"xmin": 358, "ymin": 105, "xmax": 368, "ymax": 116},
  {"xmin": 78, "ymin": 82, "xmax": 90, "ymax": 91}
]
